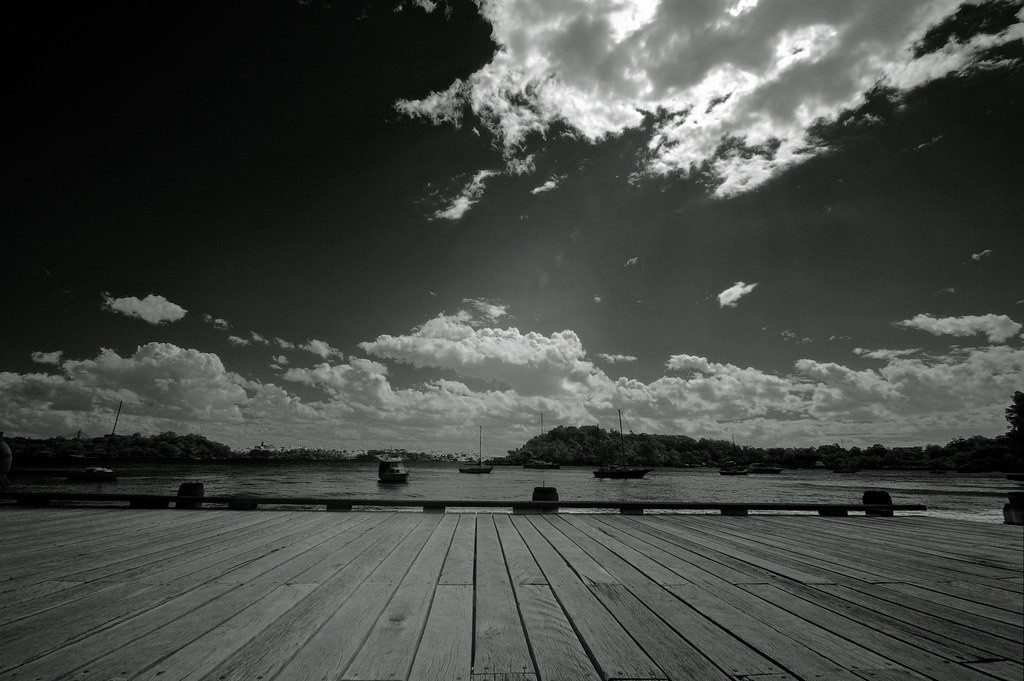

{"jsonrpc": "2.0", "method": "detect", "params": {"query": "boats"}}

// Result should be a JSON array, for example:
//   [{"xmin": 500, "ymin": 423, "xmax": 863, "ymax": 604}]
[
  {"xmin": 24, "ymin": 450, "xmax": 113, "ymax": 484},
  {"xmin": 748, "ymin": 463, "xmax": 784, "ymax": 474},
  {"xmin": 378, "ymin": 461, "xmax": 410, "ymax": 483}
]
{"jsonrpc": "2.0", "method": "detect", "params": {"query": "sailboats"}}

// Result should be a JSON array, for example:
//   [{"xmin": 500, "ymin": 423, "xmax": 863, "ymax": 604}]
[
  {"xmin": 522, "ymin": 413, "xmax": 560, "ymax": 470},
  {"xmin": 592, "ymin": 409, "xmax": 655, "ymax": 480},
  {"xmin": 459, "ymin": 426, "xmax": 494, "ymax": 474},
  {"xmin": 719, "ymin": 434, "xmax": 748, "ymax": 476}
]
